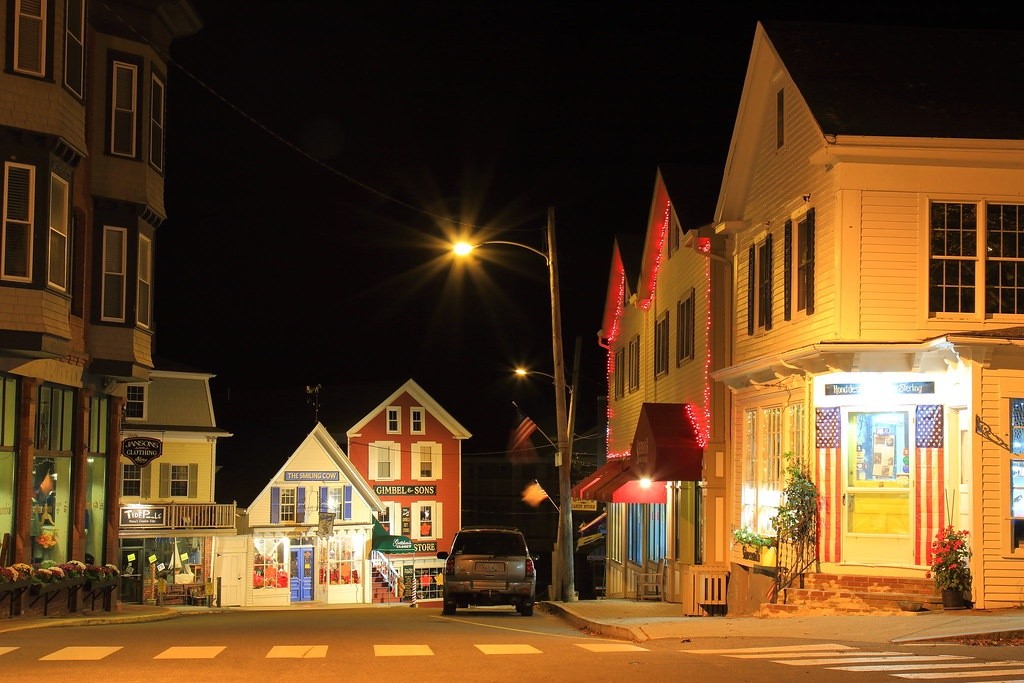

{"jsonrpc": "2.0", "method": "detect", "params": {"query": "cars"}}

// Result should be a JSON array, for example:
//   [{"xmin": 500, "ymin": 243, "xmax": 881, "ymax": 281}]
[{"xmin": 436, "ymin": 524, "xmax": 540, "ymax": 617}]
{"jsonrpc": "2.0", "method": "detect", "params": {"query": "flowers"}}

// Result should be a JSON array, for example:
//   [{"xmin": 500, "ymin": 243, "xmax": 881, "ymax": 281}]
[
  {"xmin": 83, "ymin": 564, "xmax": 121, "ymax": 582},
  {"xmin": 34, "ymin": 559, "xmax": 87, "ymax": 584},
  {"xmin": 0, "ymin": 563, "xmax": 33, "ymax": 582},
  {"xmin": 733, "ymin": 528, "xmax": 777, "ymax": 548},
  {"xmin": 926, "ymin": 525, "xmax": 971, "ymax": 590}
]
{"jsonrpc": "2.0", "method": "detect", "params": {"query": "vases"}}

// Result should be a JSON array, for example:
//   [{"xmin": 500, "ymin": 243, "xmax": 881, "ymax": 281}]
[
  {"xmin": 86, "ymin": 577, "xmax": 122, "ymax": 591},
  {"xmin": 0, "ymin": 578, "xmax": 34, "ymax": 592},
  {"xmin": 31, "ymin": 576, "xmax": 89, "ymax": 594},
  {"xmin": 941, "ymin": 588, "xmax": 968, "ymax": 611},
  {"xmin": 730, "ymin": 542, "xmax": 778, "ymax": 567}
]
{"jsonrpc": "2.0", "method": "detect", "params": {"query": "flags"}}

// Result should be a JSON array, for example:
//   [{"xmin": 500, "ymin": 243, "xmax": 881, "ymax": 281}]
[{"xmin": 507, "ymin": 404, "xmax": 541, "ymax": 464}]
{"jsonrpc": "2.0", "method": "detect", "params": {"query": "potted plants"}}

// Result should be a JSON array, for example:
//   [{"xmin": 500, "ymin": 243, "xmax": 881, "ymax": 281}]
[{"xmin": 779, "ymin": 452, "xmax": 820, "ymax": 574}]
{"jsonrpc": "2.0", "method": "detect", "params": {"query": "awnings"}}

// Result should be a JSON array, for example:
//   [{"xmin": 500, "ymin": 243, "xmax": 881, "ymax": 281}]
[{"xmin": 570, "ymin": 460, "xmax": 668, "ymax": 505}]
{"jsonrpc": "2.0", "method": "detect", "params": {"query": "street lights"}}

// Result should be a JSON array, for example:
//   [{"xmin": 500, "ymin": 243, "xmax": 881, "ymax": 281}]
[
  {"xmin": 454, "ymin": 209, "xmax": 576, "ymax": 604},
  {"xmin": 515, "ymin": 368, "xmax": 576, "ymax": 604}
]
